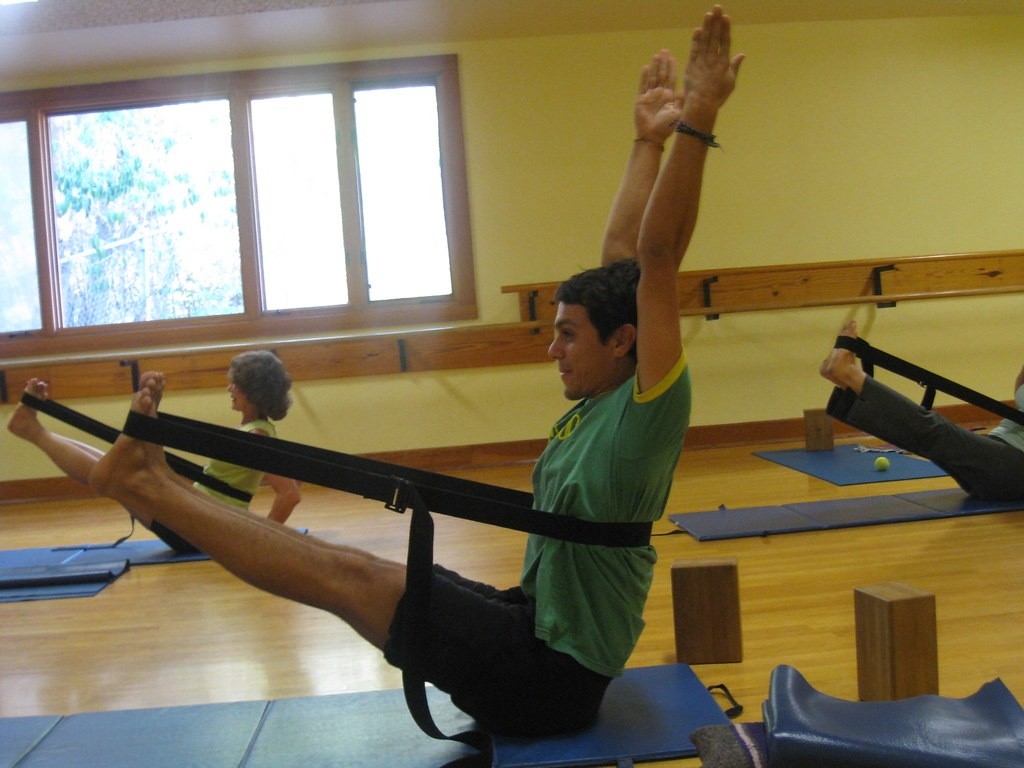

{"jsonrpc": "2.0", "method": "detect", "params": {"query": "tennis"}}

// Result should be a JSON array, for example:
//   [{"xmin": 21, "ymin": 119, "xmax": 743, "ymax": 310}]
[{"xmin": 874, "ymin": 457, "xmax": 890, "ymax": 471}]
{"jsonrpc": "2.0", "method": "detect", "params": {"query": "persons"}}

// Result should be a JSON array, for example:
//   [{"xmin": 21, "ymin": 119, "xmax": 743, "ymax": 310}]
[
  {"xmin": 8, "ymin": 350, "xmax": 305, "ymax": 556},
  {"xmin": 91, "ymin": 4, "xmax": 746, "ymax": 742},
  {"xmin": 819, "ymin": 320, "xmax": 1024, "ymax": 501}
]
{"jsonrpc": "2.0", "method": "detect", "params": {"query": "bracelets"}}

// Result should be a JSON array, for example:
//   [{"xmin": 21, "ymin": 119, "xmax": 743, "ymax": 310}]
[
  {"xmin": 667, "ymin": 119, "xmax": 719, "ymax": 148},
  {"xmin": 632, "ymin": 138, "xmax": 665, "ymax": 152}
]
